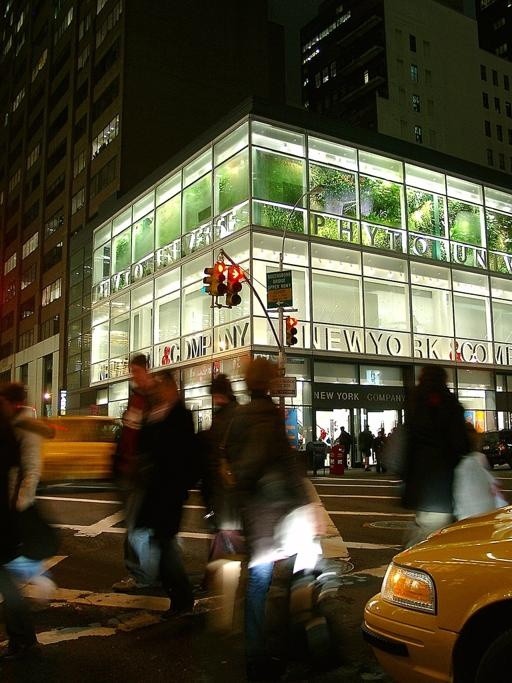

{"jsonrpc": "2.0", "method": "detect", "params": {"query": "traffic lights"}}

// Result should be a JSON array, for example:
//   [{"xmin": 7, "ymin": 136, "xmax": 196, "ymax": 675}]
[
  {"xmin": 212, "ymin": 261, "xmax": 227, "ymax": 297},
  {"xmin": 202, "ymin": 266, "xmax": 214, "ymax": 295},
  {"xmin": 226, "ymin": 264, "xmax": 242, "ymax": 307},
  {"xmin": 285, "ymin": 317, "xmax": 297, "ymax": 346}
]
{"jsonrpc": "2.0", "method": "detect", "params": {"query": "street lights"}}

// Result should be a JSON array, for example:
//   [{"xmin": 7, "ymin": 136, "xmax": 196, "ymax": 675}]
[{"xmin": 276, "ymin": 181, "xmax": 335, "ymax": 425}]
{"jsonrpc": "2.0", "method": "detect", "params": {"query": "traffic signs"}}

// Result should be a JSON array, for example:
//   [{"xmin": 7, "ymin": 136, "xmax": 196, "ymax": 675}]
[
  {"xmin": 269, "ymin": 376, "xmax": 296, "ymax": 396},
  {"xmin": 266, "ymin": 269, "xmax": 293, "ymax": 309},
  {"xmin": 275, "ymin": 367, "xmax": 285, "ymax": 375}
]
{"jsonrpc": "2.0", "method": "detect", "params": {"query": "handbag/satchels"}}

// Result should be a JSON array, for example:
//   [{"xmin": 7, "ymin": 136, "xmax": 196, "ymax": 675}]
[
  {"xmin": 381, "ymin": 422, "xmax": 411, "ymax": 472},
  {"xmin": 14, "ymin": 502, "xmax": 58, "ymax": 561}
]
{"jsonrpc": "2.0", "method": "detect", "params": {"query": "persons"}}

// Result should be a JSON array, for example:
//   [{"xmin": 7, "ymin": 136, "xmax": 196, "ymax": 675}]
[
  {"xmin": 359, "ymin": 425, "xmax": 396, "ymax": 473},
  {"xmin": 401, "ymin": 365, "xmax": 509, "ymax": 549},
  {"xmin": 336, "ymin": 426, "xmax": 351, "ymax": 470},
  {"xmin": 113, "ymin": 354, "xmax": 386, "ymax": 682},
  {"xmin": 0, "ymin": 383, "xmax": 58, "ymax": 663}
]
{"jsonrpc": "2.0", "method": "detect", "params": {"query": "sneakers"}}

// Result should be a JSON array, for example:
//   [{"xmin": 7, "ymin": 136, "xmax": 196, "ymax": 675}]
[
  {"xmin": 365, "ymin": 467, "xmax": 371, "ymax": 471},
  {"xmin": 111, "ymin": 573, "xmax": 151, "ymax": 591}
]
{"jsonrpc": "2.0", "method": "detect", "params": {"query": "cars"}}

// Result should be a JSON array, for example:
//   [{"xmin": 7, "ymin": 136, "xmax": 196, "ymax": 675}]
[
  {"xmin": 480, "ymin": 427, "xmax": 511, "ymax": 467},
  {"xmin": 358, "ymin": 501, "xmax": 511, "ymax": 681},
  {"xmin": 36, "ymin": 414, "xmax": 124, "ymax": 486}
]
